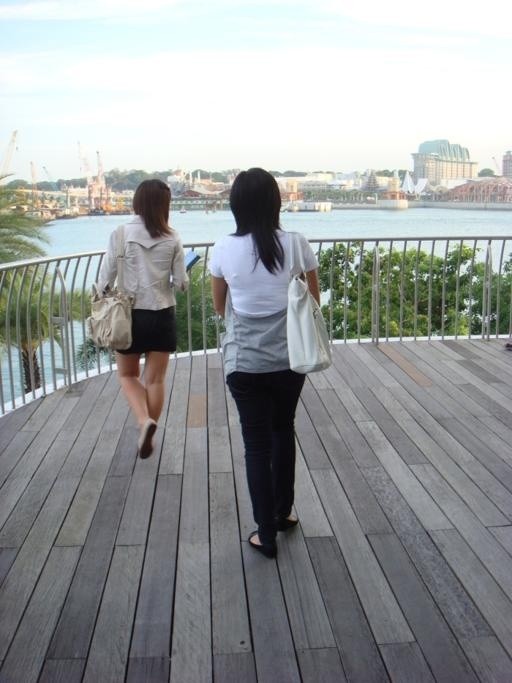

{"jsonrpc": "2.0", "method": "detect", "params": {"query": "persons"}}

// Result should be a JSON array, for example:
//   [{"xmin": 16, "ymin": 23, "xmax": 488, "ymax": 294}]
[
  {"xmin": 208, "ymin": 167, "xmax": 320, "ymax": 559},
  {"xmin": 97, "ymin": 178, "xmax": 190, "ymax": 458}
]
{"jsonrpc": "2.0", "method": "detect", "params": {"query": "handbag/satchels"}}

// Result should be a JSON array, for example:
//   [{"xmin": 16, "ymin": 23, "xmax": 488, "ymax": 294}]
[
  {"xmin": 86, "ymin": 282, "xmax": 134, "ymax": 350},
  {"xmin": 286, "ymin": 276, "xmax": 333, "ymax": 373}
]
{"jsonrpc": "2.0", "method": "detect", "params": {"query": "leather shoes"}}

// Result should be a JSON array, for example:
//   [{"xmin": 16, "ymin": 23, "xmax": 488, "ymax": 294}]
[
  {"xmin": 138, "ymin": 418, "xmax": 157, "ymax": 458},
  {"xmin": 248, "ymin": 530, "xmax": 277, "ymax": 559},
  {"xmin": 277, "ymin": 516, "xmax": 299, "ymax": 531}
]
